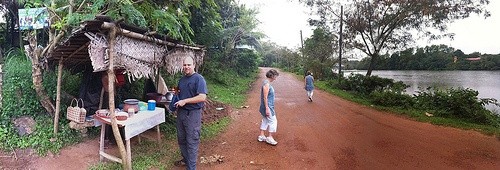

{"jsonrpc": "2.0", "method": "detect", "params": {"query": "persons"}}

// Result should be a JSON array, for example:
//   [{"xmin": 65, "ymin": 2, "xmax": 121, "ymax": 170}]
[
  {"xmin": 304, "ymin": 71, "xmax": 314, "ymax": 101},
  {"xmin": 174, "ymin": 56, "xmax": 208, "ymax": 170},
  {"xmin": 101, "ymin": 70, "xmax": 126, "ymax": 143},
  {"xmin": 259, "ymin": 69, "xmax": 278, "ymax": 145}
]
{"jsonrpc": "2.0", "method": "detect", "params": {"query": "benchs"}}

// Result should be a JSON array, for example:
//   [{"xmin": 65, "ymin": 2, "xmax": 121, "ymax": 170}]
[{"xmin": 69, "ymin": 118, "xmax": 100, "ymax": 142}]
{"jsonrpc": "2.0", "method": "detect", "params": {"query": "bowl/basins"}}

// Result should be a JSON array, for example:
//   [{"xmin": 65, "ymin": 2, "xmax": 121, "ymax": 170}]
[{"xmin": 117, "ymin": 114, "xmax": 127, "ymax": 120}]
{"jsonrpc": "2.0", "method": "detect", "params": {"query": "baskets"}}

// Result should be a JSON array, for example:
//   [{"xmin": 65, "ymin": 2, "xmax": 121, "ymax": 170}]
[{"xmin": 67, "ymin": 97, "xmax": 87, "ymax": 123}]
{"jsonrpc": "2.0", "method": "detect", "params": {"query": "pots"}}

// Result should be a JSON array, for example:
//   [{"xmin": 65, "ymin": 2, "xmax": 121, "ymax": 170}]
[{"xmin": 124, "ymin": 98, "xmax": 139, "ymax": 114}]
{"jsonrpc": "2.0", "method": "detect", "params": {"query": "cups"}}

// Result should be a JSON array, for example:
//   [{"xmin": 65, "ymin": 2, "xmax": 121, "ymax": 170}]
[
  {"xmin": 174, "ymin": 86, "xmax": 180, "ymax": 94},
  {"xmin": 139, "ymin": 102, "xmax": 144, "ymax": 111},
  {"xmin": 148, "ymin": 99, "xmax": 156, "ymax": 110},
  {"xmin": 128, "ymin": 108, "xmax": 134, "ymax": 117},
  {"xmin": 144, "ymin": 103, "xmax": 148, "ymax": 110}
]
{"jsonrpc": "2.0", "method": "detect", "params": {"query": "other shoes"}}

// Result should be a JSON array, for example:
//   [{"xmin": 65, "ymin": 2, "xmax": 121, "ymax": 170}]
[
  {"xmin": 266, "ymin": 135, "xmax": 277, "ymax": 145},
  {"xmin": 258, "ymin": 135, "xmax": 267, "ymax": 142},
  {"xmin": 174, "ymin": 159, "xmax": 187, "ymax": 167},
  {"xmin": 308, "ymin": 96, "xmax": 312, "ymax": 102}
]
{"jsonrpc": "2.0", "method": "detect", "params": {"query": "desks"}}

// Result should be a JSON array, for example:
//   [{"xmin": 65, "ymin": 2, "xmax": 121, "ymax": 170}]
[{"xmin": 94, "ymin": 107, "xmax": 165, "ymax": 170}]
